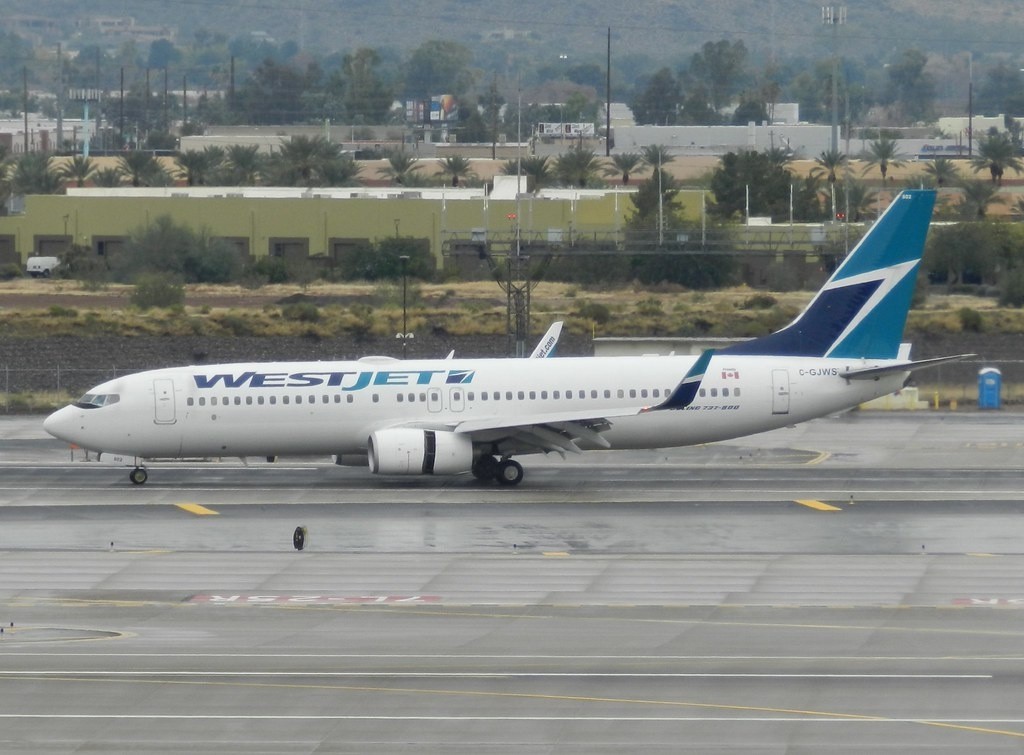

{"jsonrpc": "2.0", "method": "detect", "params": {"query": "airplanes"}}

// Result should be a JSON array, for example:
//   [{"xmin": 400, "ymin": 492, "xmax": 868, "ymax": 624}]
[{"xmin": 43, "ymin": 190, "xmax": 977, "ymax": 487}]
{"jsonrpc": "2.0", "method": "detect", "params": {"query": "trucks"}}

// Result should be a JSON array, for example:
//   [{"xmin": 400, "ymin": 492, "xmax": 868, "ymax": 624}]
[{"xmin": 26, "ymin": 256, "xmax": 68, "ymax": 278}]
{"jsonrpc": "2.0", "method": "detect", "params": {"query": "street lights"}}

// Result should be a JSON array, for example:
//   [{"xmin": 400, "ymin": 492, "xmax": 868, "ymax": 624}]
[
  {"xmin": 396, "ymin": 255, "xmax": 413, "ymax": 358},
  {"xmin": 560, "ymin": 55, "xmax": 568, "ymax": 146},
  {"xmin": 822, "ymin": 5, "xmax": 847, "ymax": 154}
]
